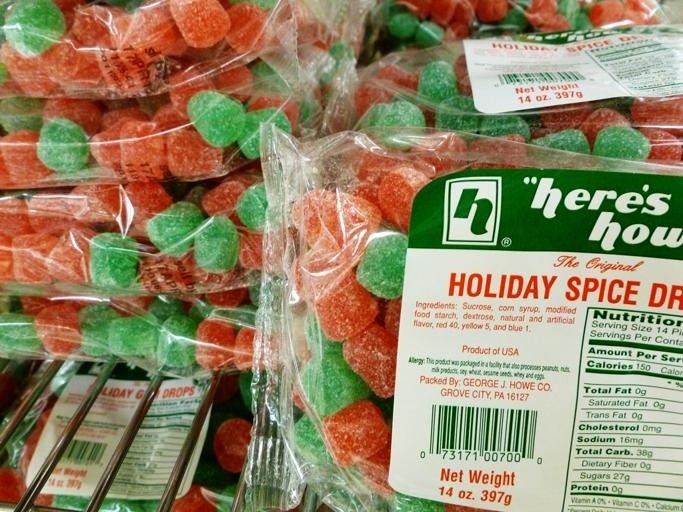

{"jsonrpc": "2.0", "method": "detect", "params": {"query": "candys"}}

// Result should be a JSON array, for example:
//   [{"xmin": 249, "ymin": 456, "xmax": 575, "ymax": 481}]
[{"xmin": 0, "ymin": 0, "xmax": 683, "ymax": 512}]
